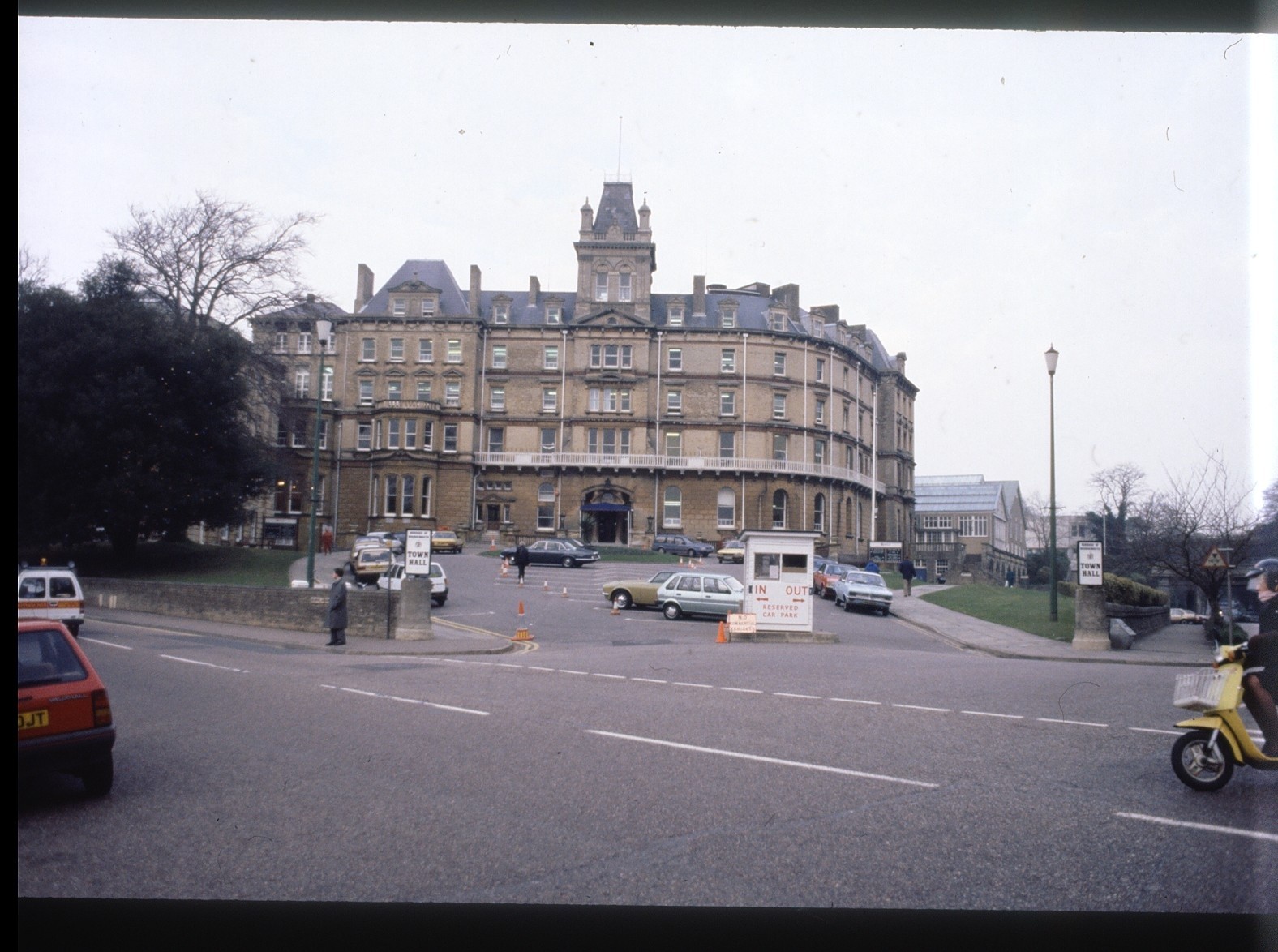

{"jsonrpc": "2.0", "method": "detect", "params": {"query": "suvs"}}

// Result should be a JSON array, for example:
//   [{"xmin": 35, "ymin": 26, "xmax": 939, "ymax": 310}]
[
  {"xmin": 656, "ymin": 572, "xmax": 747, "ymax": 620},
  {"xmin": 18, "ymin": 619, "xmax": 116, "ymax": 811},
  {"xmin": 18, "ymin": 558, "xmax": 85, "ymax": 637},
  {"xmin": 376, "ymin": 561, "xmax": 449, "ymax": 607}
]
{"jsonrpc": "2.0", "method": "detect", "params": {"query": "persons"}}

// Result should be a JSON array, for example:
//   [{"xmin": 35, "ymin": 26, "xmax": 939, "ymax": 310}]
[
  {"xmin": 321, "ymin": 526, "xmax": 334, "ymax": 556},
  {"xmin": 899, "ymin": 554, "xmax": 917, "ymax": 597},
  {"xmin": 1216, "ymin": 559, "xmax": 1278, "ymax": 757},
  {"xmin": 865, "ymin": 557, "xmax": 881, "ymax": 573},
  {"xmin": 326, "ymin": 566, "xmax": 347, "ymax": 648},
  {"xmin": 1006, "ymin": 566, "xmax": 1016, "ymax": 587},
  {"xmin": 515, "ymin": 540, "xmax": 532, "ymax": 584}
]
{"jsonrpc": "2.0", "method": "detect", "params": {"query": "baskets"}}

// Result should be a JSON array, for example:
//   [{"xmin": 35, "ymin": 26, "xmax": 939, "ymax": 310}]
[{"xmin": 1172, "ymin": 666, "xmax": 1230, "ymax": 712}]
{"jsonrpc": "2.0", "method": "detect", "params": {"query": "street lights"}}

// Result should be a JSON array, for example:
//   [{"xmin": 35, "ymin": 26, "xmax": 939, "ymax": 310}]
[
  {"xmin": 307, "ymin": 311, "xmax": 332, "ymax": 592},
  {"xmin": 1043, "ymin": 341, "xmax": 1061, "ymax": 624}
]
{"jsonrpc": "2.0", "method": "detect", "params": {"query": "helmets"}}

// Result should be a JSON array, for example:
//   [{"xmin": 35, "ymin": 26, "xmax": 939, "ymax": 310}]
[{"xmin": 1244, "ymin": 558, "xmax": 1278, "ymax": 592}]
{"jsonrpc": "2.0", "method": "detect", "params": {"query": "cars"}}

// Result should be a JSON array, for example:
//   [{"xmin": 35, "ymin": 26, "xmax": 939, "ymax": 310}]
[
  {"xmin": 812, "ymin": 555, "xmax": 837, "ymax": 575},
  {"xmin": 816, "ymin": 563, "xmax": 859, "ymax": 600},
  {"xmin": 630, "ymin": 533, "xmax": 714, "ymax": 556},
  {"xmin": 428, "ymin": 527, "xmax": 463, "ymax": 553},
  {"xmin": 834, "ymin": 570, "xmax": 892, "ymax": 615},
  {"xmin": 368, "ymin": 531, "xmax": 404, "ymax": 556},
  {"xmin": 717, "ymin": 540, "xmax": 747, "ymax": 563},
  {"xmin": 602, "ymin": 571, "xmax": 681, "ymax": 609},
  {"xmin": 499, "ymin": 539, "xmax": 600, "ymax": 569},
  {"xmin": 350, "ymin": 536, "xmax": 400, "ymax": 583}
]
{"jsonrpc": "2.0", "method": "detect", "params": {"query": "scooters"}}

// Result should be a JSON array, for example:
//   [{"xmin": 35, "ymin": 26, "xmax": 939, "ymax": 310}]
[{"xmin": 1170, "ymin": 641, "xmax": 1278, "ymax": 791}]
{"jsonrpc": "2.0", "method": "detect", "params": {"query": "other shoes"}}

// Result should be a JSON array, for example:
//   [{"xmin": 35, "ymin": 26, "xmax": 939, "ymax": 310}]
[
  {"xmin": 325, "ymin": 642, "xmax": 345, "ymax": 646},
  {"xmin": 904, "ymin": 595, "xmax": 907, "ymax": 597},
  {"xmin": 908, "ymin": 594, "xmax": 911, "ymax": 596}
]
